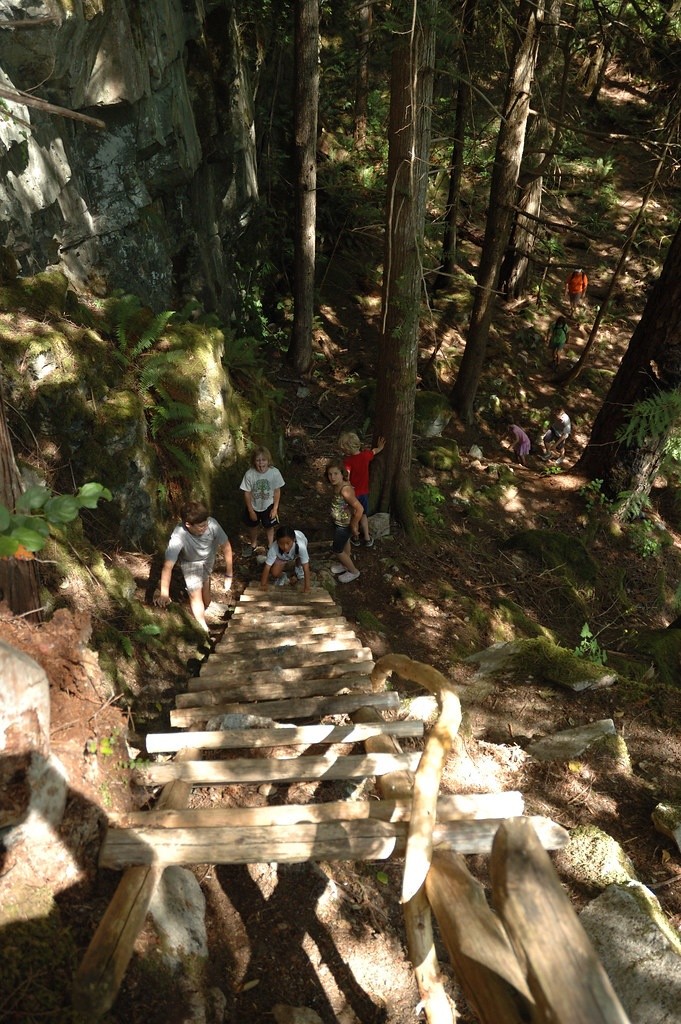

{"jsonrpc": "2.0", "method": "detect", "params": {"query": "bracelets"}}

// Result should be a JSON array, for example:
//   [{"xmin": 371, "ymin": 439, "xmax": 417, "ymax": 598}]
[{"xmin": 224, "ymin": 574, "xmax": 232, "ymax": 579}]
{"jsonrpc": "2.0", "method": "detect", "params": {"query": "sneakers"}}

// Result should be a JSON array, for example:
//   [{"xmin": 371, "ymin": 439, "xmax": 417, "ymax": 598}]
[
  {"xmin": 295, "ymin": 565, "xmax": 305, "ymax": 580},
  {"xmin": 274, "ymin": 572, "xmax": 287, "ymax": 586}
]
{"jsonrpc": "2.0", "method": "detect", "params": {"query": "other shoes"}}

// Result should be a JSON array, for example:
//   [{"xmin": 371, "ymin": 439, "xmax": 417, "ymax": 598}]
[
  {"xmin": 542, "ymin": 453, "xmax": 551, "ymax": 460},
  {"xmin": 240, "ymin": 547, "xmax": 256, "ymax": 556},
  {"xmin": 555, "ymin": 456, "xmax": 565, "ymax": 464},
  {"xmin": 365, "ymin": 535, "xmax": 374, "ymax": 546},
  {"xmin": 206, "ymin": 629, "xmax": 216, "ymax": 642},
  {"xmin": 349, "ymin": 536, "xmax": 360, "ymax": 546}
]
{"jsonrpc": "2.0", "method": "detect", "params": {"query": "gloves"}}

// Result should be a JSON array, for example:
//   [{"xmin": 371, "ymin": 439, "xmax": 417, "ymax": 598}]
[{"xmin": 223, "ymin": 576, "xmax": 232, "ymax": 592}]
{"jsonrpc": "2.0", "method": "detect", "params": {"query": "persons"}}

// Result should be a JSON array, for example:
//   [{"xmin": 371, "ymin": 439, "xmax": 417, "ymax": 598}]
[
  {"xmin": 541, "ymin": 402, "xmax": 572, "ymax": 464},
  {"xmin": 324, "ymin": 462, "xmax": 366, "ymax": 583},
  {"xmin": 565, "ymin": 267, "xmax": 588, "ymax": 313},
  {"xmin": 153, "ymin": 500, "xmax": 233, "ymax": 640},
  {"xmin": 544, "ymin": 316, "xmax": 571, "ymax": 366},
  {"xmin": 261, "ymin": 525, "xmax": 314, "ymax": 596},
  {"xmin": 238, "ymin": 446, "xmax": 285, "ymax": 556},
  {"xmin": 339, "ymin": 431, "xmax": 387, "ymax": 547},
  {"xmin": 504, "ymin": 415, "xmax": 531, "ymax": 466}
]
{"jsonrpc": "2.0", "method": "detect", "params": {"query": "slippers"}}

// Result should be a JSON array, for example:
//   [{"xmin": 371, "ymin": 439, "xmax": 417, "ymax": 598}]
[
  {"xmin": 338, "ymin": 570, "xmax": 362, "ymax": 583},
  {"xmin": 331, "ymin": 563, "xmax": 349, "ymax": 574}
]
